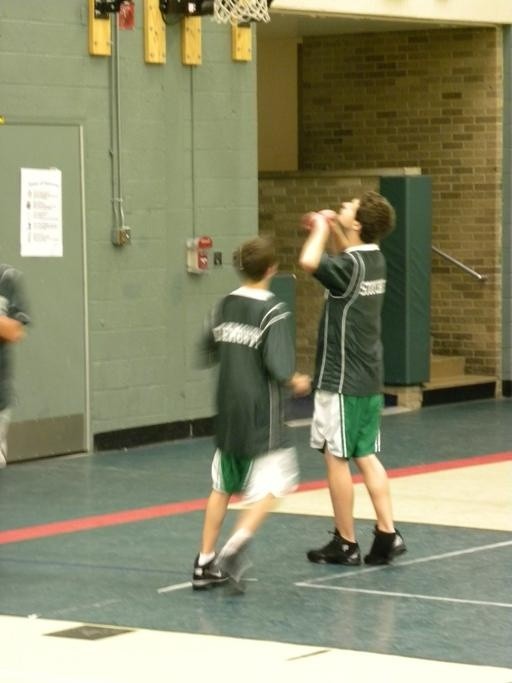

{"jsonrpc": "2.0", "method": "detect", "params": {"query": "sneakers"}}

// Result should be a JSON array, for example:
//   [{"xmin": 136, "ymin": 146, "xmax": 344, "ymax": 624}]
[
  {"xmin": 364, "ymin": 523, "xmax": 408, "ymax": 565},
  {"xmin": 306, "ymin": 528, "xmax": 363, "ymax": 566},
  {"xmin": 190, "ymin": 537, "xmax": 253, "ymax": 595}
]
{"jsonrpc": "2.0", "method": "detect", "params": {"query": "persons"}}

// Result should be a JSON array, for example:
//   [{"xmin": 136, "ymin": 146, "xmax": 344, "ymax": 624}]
[
  {"xmin": 1, "ymin": 261, "xmax": 28, "ymax": 471},
  {"xmin": 300, "ymin": 192, "xmax": 407, "ymax": 564},
  {"xmin": 192, "ymin": 239, "xmax": 312, "ymax": 592}
]
